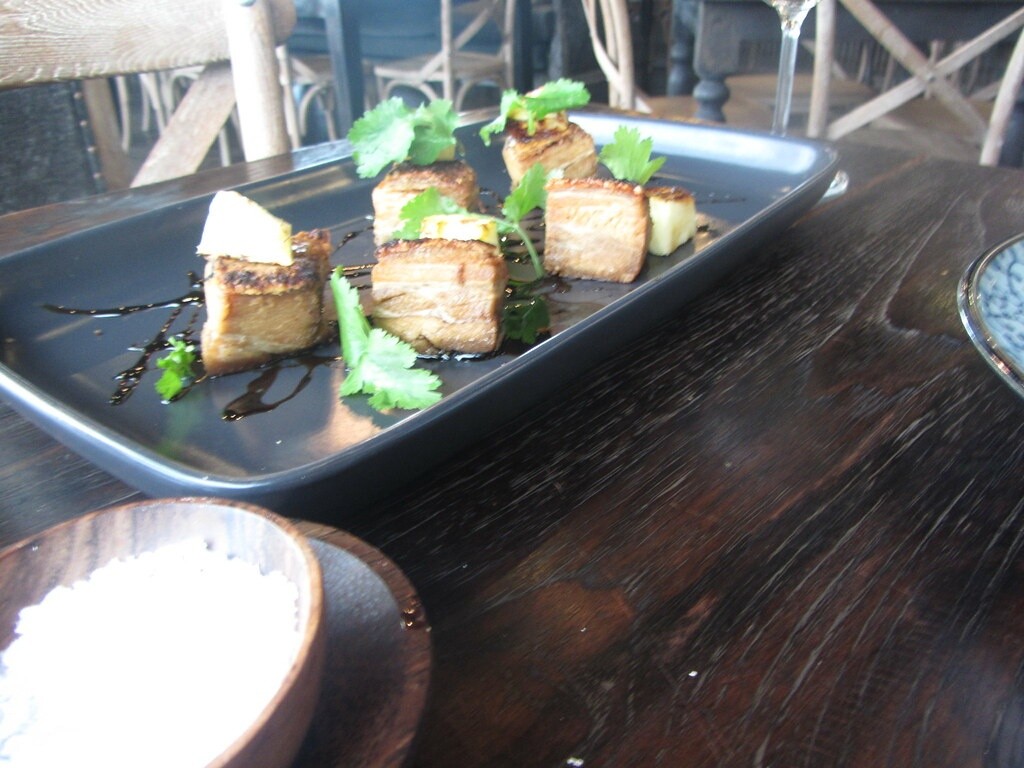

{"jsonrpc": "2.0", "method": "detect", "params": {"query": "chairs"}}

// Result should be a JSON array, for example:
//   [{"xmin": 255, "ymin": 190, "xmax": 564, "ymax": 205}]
[{"xmin": 0, "ymin": 0, "xmax": 1024, "ymax": 211}]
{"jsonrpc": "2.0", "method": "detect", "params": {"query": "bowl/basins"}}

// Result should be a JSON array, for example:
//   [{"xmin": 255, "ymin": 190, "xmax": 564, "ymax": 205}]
[{"xmin": 0, "ymin": 496, "xmax": 325, "ymax": 768}]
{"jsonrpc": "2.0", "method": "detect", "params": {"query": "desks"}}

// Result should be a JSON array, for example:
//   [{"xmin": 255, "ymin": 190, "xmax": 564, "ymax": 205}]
[{"xmin": 1, "ymin": 88, "xmax": 1024, "ymax": 767}]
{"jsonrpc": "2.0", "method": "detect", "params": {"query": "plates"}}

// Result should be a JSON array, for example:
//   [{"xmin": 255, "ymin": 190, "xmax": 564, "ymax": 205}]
[
  {"xmin": 286, "ymin": 517, "xmax": 431, "ymax": 768},
  {"xmin": 957, "ymin": 230, "xmax": 1024, "ymax": 402}
]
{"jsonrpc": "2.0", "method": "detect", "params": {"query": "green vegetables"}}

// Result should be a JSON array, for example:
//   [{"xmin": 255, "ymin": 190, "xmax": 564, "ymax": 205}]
[
  {"xmin": 330, "ymin": 268, "xmax": 442, "ymax": 413},
  {"xmin": 155, "ymin": 337, "xmax": 195, "ymax": 402},
  {"xmin": 345, "ymin": 78, "xmax": 666, "ymax": 275},
  {"xmin": 501, "ymin": 295, "xmax": 552, "ymax": 346}
]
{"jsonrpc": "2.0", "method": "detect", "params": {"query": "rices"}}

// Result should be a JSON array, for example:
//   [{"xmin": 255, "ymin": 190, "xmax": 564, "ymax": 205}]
[{"xmin": 0, "ymin": 540, "xmax": 306, "ymax": 768}]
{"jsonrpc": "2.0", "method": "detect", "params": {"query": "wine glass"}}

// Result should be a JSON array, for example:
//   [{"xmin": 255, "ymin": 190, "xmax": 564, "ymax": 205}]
[{"xmin": 759, "ymin": 1, "xmax": 849, "ymax": 201}]
